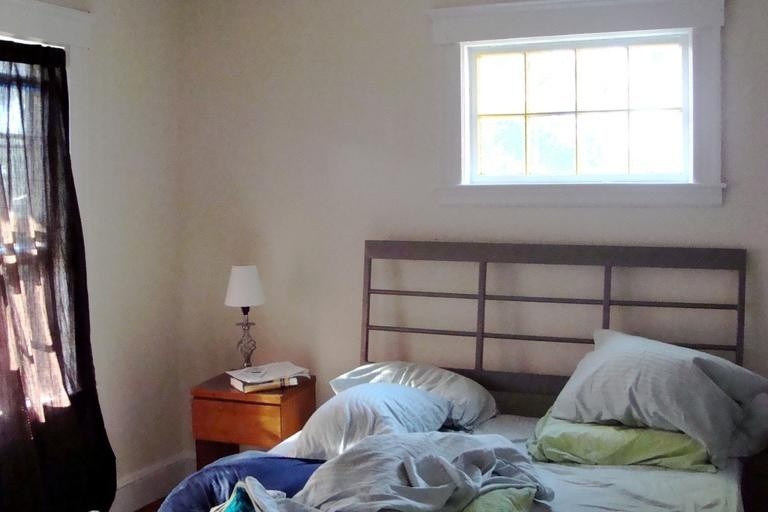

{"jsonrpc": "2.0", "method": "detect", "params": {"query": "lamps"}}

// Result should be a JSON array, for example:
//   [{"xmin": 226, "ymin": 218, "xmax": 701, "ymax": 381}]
[{"xmin": 223, "ymin": 263, "xmax": 266, "ymax": 366}]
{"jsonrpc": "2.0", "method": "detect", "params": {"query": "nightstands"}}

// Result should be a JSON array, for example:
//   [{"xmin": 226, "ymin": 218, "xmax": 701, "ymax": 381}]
[{"xmin": 190, "ymin": 367, "xmax": 318, "ymax": 472}]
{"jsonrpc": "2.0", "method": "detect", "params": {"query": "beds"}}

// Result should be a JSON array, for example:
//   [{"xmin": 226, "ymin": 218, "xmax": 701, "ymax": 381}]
[{"xmin": 268, "ymin": 239, "xmax": 748, "ymax": 511}]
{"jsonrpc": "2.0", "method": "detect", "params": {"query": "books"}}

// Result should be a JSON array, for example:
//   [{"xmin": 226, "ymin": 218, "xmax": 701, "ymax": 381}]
[
  {"xmin": 224, "ymin": 359, "xmax": 312, "ymax": 383},
  {"xmin": 230, "ymin": 377, "xmax": 298, "ymax": 394}
]
